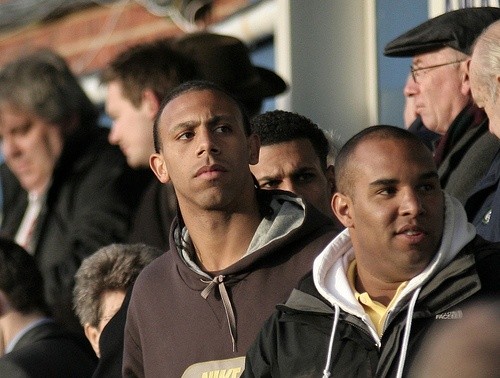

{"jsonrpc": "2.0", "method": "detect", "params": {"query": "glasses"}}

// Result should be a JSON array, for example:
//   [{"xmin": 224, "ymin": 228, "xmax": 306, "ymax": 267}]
[{"xmin": 410, "ymin": 60, "xmax": 465, "ymax": 83}]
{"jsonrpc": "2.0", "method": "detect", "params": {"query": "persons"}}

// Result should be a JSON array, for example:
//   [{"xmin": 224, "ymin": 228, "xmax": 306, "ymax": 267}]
[
  {"xmin": 72, "ymin": 243, "xmax": 167, "ymax": 377},
  {"xmin": 382, "ymin": 7, "xmax": 500, "ymax": 206},
  {"xmin": 463, "ymin": 18, "xmax": 500, "ymax": 243},
  {"xmin": 122, "ymin": 80, "xmax": 347, "ymax": 378},
  {"xmin": 0, "ymin": 236, "xmax": 101, "ymax": 378},
  {"xmin": 245, "ymin": 111, "xmax": 348, "ymax": 233},
  {"xmin": 98, "ymin": 38, "xmax": 183, "ymax": 253},
  {"xmin": 403, "ymin": 71, "xmax": 445, "ymax": 153},
  {"xmin": 1, "ymin": 46, "xmax": 135, "ymax": 343},
  {"xmin": 240, "ymin": 128, "xmax": 500, "ymax": 378},
  {"xmin": 130, "ymin": 32, "xmax": 290, "ymax": 253}
]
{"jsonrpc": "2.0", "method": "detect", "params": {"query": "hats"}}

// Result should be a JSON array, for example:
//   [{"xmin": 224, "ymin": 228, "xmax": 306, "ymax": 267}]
[
  {"xmin": 171, "ymin": 33, "xmax": 289, "ymax": 104},
  {"xmin": 382, "ymin": 7, "xmax": 500, "ymax": 56}
]
